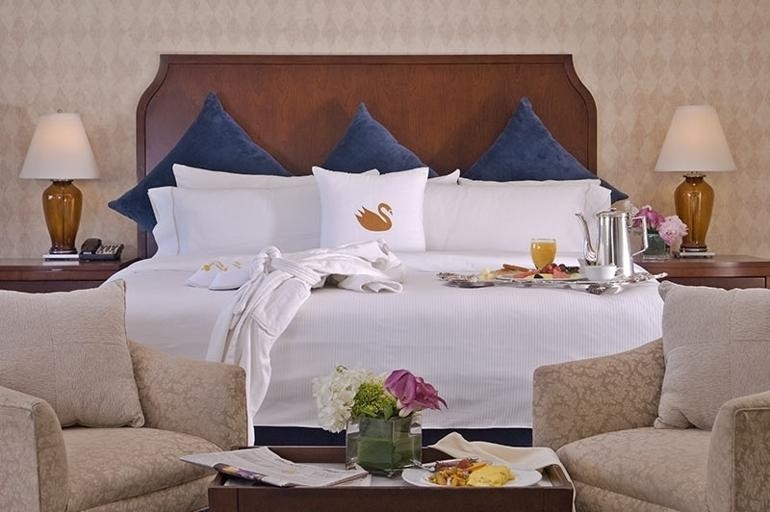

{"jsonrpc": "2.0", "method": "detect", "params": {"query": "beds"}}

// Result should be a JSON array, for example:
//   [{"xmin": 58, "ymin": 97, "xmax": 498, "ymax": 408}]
[{"xmin": 95, "ymin": 52, "xmax": 665, "ymax": 444}]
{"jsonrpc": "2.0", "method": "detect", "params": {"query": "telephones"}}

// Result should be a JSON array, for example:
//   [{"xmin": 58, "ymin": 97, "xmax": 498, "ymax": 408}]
[{"xmin": 79, "ymin": 238, "xmax": 123, "ymax": 260}]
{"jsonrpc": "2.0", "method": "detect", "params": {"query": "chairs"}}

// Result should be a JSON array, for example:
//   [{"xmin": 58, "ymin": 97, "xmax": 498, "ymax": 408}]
[
  {"xmin": 0, "ymin": 289, "xmax": 248, "ymax": 511},
  {"xmin": 531, "ymin": 338, "xmax": 770, "ymax": 512}
]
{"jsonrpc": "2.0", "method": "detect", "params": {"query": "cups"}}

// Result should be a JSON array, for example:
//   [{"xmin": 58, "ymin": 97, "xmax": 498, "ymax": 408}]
[
  {"xmin": 530, "ymin": 238, "xmax": 558, "ymax": 271},
  {"xmin": 581, "ymin": 265, "xmax": 624, "ymax": 283}
]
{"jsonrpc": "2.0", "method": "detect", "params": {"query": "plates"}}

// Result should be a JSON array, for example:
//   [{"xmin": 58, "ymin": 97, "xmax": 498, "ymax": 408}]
[
  {"xmin": 570, "ymin": 280, "xmax": 629, "ymax": 291},
  {"xmin": 401, "ymin": 458, "xmax": 543, "ymax": 488}
]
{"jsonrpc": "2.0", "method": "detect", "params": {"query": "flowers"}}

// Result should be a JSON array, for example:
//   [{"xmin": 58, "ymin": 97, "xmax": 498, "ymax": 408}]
[
  {"xmin": 311, "ymin": 362, "xmax": 449, "ymax": 470},
  {"xmin": 631, "ymin": 203, "xmax": 689, "ymax": 247}
]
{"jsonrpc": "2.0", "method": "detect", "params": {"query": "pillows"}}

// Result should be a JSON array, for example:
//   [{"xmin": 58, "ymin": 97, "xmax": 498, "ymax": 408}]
[
  {"xmin": 1, "ymin": 278, "xmax": 146, "ymax": 429},
  {"xmin": 652, "ymin": 280, "xmax": 770, "ymax": 430}
]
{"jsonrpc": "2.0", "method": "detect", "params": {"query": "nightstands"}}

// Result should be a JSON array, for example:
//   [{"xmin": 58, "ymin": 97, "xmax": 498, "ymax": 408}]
[
  {"xmin": 633, "ymin": 254, "xmax": 770, "ymax": 291},
  {"xmin": 0, "ymin": 257, "xmax": 141, "ymax": 294}
]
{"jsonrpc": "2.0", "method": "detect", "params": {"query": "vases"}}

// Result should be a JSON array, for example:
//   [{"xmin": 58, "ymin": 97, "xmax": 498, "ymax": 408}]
[
  {"xmin": 641, "ymin": 229, "xmax": 664, "ymax": 255},
  {"xmin": 345, "ymin": 415, "xmax": 423, "ymax": 479}
]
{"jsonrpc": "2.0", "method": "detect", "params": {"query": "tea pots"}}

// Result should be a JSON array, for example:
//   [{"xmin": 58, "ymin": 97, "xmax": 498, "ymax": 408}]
[{"xmin": 574, "ymin": 209, "xmax": 648, "ymax": 280}]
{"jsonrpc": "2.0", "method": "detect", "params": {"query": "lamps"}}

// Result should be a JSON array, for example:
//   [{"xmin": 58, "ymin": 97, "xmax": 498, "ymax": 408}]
[
  {"xmin": 654, "ymin": 104, "xmax": 737, "ymax": 257},
  {"xmin": 18, "ymin": 108, "xmax": 101, "ymax": 258}
]
